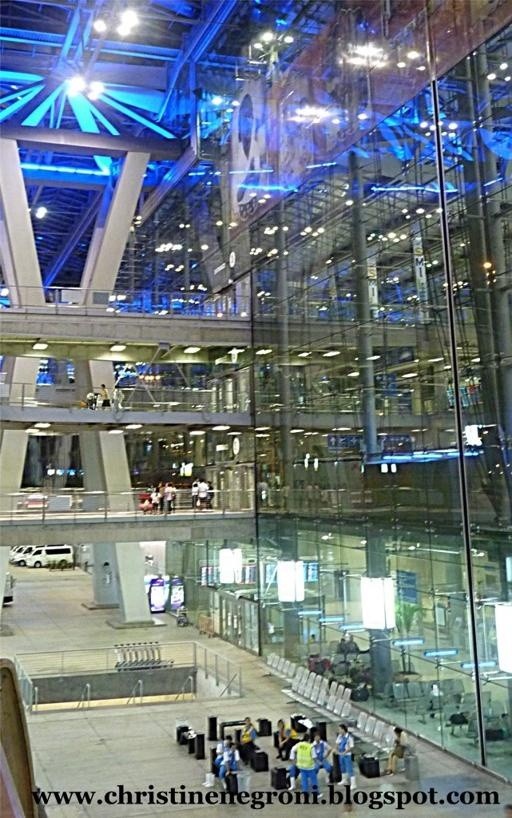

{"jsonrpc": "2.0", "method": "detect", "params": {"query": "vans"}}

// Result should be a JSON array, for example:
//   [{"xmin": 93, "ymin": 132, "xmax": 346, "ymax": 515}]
[{"xmin": 9, "ymin": 544, "xmax": 73, "ymax": 568}]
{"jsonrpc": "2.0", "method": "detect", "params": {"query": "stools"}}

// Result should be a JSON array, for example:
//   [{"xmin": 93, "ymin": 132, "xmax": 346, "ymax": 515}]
[{"xmin": 177, "ymin": 716, "xmax": 268, "ymax": 792}]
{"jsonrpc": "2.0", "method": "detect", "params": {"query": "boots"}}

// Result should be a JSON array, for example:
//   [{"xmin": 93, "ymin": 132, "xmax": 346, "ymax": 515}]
[
  {"xmin": 286, "ymin": 777, "xmax": 297, "ymax": 791},
  {"xmin": 202, "ymin": 772, "xmax": 216, "ymax": 788},
  {"xmin": 348, "ymin": 776, "xmax": 356, "ymax": 790},
  {"xmin": 337, "ymin": 774, "xmax": 348, "ymax": 786}
]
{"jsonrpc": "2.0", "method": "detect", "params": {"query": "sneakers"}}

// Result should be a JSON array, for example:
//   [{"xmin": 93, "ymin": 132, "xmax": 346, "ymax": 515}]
[{"xmin": 313, "ymin": 793, "xmax": 324, "ymax": 799}]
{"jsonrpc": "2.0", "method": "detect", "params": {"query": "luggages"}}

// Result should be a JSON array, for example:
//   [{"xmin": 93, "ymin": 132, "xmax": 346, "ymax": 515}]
[
  {"xmin": 256, "ymin": 718, "xmax": 272, "ymax": 737},
  {"xmin": 269, "ymin": 765, "xmax": 287, "ymax": 791},
  {"xmin": 176, "ymin": 725, "xmax": 190, "ymax": 743},
  {"xmin": 249, "ymin": 751, "xmax": 270, "ymax": 773},
  {"xmin": 358, "ymin": 752, "xmax": 380, "ymax": 778},
  {"xmin": 225, "ymin": 772, "xmax": 239, "ymax": 795}
]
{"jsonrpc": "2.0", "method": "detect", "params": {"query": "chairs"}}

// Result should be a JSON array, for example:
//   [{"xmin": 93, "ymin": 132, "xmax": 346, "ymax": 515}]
[{"xmin": 261, "ymin": 646, "xmax": 411, "ymax": 773}]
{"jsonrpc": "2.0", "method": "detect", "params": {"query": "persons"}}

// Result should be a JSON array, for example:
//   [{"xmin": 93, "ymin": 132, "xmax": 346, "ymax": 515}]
[
  {"xmin": 83, "ymin": 391, "xmax": 102, "ymax": 411},
  {"xmin": 211, "ymin": 718, "xmax": 408, "ymax": 797},
  {"xmin": 138, "ymin": 476, "xmax": 215, "ymax": 514},
  {"xmin": 99, "ymin": 383, "xmax": 110, "ymax": 409},
  {"xmin": 307, "ymin": 633, "xmax": 371, "ymax": 702},
  {"xmin": 110, "ymin": 385, "xmax": 126, "ymax": 412}
]
